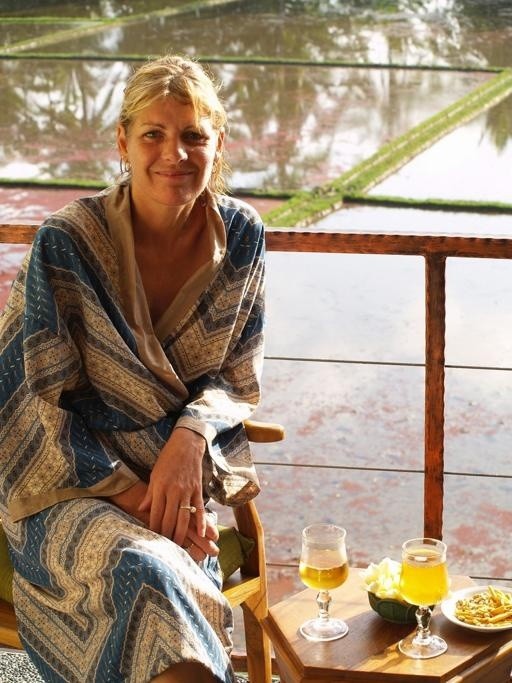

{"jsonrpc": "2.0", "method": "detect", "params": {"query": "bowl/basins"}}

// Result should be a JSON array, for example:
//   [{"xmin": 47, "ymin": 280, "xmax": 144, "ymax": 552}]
[{"xmin": 364, "ymin": 590, "xmax": 436, "ymax": 626}]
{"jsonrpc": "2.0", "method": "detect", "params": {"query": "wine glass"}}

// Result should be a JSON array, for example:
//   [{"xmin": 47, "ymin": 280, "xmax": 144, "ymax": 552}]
[
  {"xmin": 396, "ymin": 536, "xmax": 449, "ymax": 661},
  {"xmin": 296, "ymin": 522, "xmax": 350, "ymax": 644}
]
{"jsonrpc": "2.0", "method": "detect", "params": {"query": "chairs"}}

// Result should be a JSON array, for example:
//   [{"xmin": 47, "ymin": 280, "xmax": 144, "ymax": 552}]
[{"xmin": 1, "ymin": 418, "xmax": 285, "ymax": 682}]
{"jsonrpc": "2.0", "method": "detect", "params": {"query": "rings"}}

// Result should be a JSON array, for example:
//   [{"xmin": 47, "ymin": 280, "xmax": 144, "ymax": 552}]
[{"xmin": 178, "ymin": 504, "xmax": 198, "ymax": 513}]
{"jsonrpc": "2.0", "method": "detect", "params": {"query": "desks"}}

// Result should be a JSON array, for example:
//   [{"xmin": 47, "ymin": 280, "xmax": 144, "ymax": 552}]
[{"xmin": 257, "ymin": 566, "xmax": 512, "ymax": 683}]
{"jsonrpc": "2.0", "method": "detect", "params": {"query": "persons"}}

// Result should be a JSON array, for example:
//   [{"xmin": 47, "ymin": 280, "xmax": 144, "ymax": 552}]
[{"xmin": 2, "ymin": 52, "xmax": 268, "ymax": 682}]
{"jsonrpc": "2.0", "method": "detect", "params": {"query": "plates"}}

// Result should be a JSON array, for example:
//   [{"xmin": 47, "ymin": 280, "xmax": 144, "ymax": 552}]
[{"xmin": 439, "ymin": 583, "xmax": 512, "ymax": 634}]
